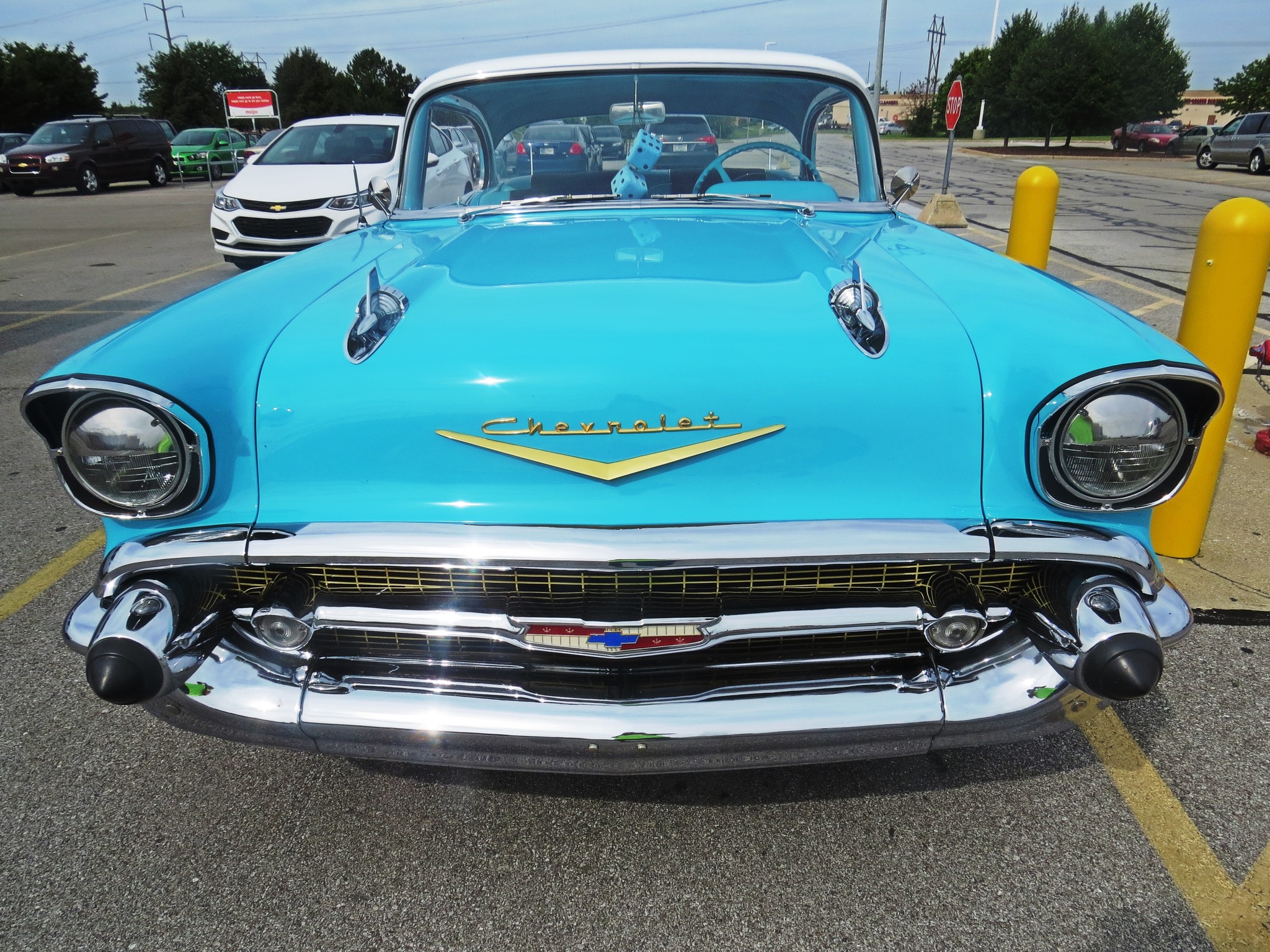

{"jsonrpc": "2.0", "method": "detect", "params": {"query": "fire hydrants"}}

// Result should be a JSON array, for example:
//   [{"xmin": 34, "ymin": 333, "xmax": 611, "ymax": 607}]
[{"xmin": 1247, "ymin": 339, "xmax": 1270, "ymax": 456}]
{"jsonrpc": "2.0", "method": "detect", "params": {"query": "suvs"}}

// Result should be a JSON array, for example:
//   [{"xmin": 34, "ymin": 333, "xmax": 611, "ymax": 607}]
[
  {"xmin": 0, "ymin": 114, "xmax": 174, "ymax": 197},
  {"xmin": 632, "ymin": 113, "xmax": 719, "ymax": 181}
]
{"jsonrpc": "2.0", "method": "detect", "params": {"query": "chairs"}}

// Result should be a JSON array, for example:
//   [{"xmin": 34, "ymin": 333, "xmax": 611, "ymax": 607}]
[{"xmin": 493, "ymin": 167, "xmax": 793, "ymax": 202}]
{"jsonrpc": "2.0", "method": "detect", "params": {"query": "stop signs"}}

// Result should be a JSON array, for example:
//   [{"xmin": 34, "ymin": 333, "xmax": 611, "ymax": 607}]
[{"xmin": 945, "ymin": 80, "xmax": 963, "ymax": 131}]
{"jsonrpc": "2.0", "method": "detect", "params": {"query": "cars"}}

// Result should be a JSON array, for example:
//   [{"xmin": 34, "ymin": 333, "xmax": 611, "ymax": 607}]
[
  {"xmin": 1196, "ymin": 112, "xmax": 1270, "ymax": 175},
  {"xmin": 516, "ymin": 120, "xmax": 604, "ymax": 177},
  {"xmin": 18, "ymin": 46, "xmax": 1227, "ymax": 775},
  {"xmin": 1111, "ymin": 121, "xmax": 1179, "ymax": 153},
  {"xmin": 210, "ymin": 113, "xmax": 476, "ymax": 270},
  {"xmin": 156, "ymin": 118, "xmax": 178, "ymax": 141},
  {"xmin": 591, "ymin": 125, "xmax": 628, "ymax": 160},
  {"xmin": 0, "ymin": 133, "xmax": 49, "ymax": 154},
  {"xmin": 1166, "ymin": 121, "xmax": 1197, "ymax": 134},
  {"xmin": 878, "ymin": 116, "xmax": 909, "ymax": 136},
  {"xmin": 244, "ymin": 128, "xmax": 332, "ymax": 166},
  {"xmin": 1166, "ymin": 125, "xmax": 1225, "ymax": 155},
  {"xmin": 168, "ymin": 127, "xmax": 257, "ymax": 181},
  {"xmin": 767, "ymin": 123, "xmax": 780, "ymax": 132},
  {"xmin": 438, "ymin": 125, "xmax": 518, "ymax": 183}
]
